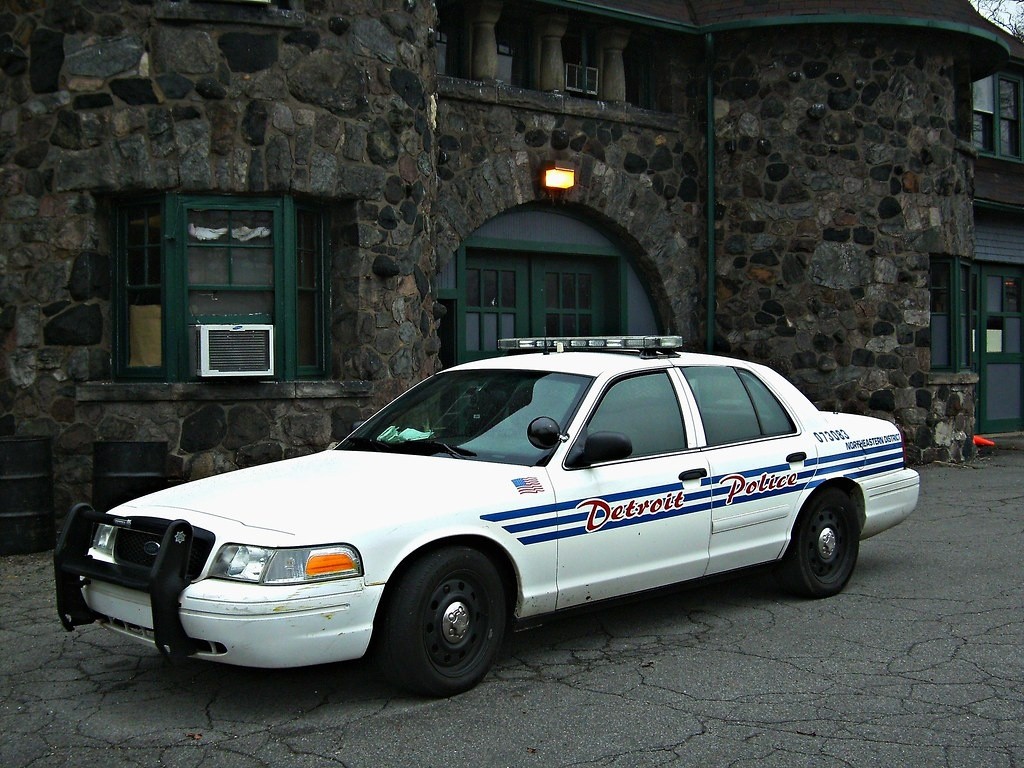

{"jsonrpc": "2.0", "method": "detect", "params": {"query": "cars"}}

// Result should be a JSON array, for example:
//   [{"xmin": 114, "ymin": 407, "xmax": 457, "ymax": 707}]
[{"xmin": 52, "ymin": 336, "xmax": 922, "ymax": 705}]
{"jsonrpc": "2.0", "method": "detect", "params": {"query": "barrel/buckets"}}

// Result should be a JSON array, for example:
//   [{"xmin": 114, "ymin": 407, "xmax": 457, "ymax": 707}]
[
  {"xmin": 94, "ymin": 442, "xmax": 168, "ymax": 513},
  {"xmin": 0, "ymin": 435, "xmax": 54, "ymax": 554}
]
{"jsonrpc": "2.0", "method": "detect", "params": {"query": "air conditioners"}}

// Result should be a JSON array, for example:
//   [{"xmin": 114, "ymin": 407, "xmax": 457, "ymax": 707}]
[{"xmin": 188, "ymin": 324, "xmax": 274, "ymax": 377}]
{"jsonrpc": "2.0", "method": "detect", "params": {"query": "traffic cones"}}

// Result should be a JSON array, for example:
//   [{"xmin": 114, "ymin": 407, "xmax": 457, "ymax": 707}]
[{"xmin": 973, "ymin": 435, "xmax": 996, "ymax": 448}]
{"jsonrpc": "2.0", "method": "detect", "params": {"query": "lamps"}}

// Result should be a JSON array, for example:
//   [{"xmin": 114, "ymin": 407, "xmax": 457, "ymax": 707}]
[{"xmin": 545, "ymin": 160, "xmax": 575, "ymax": 188}]
